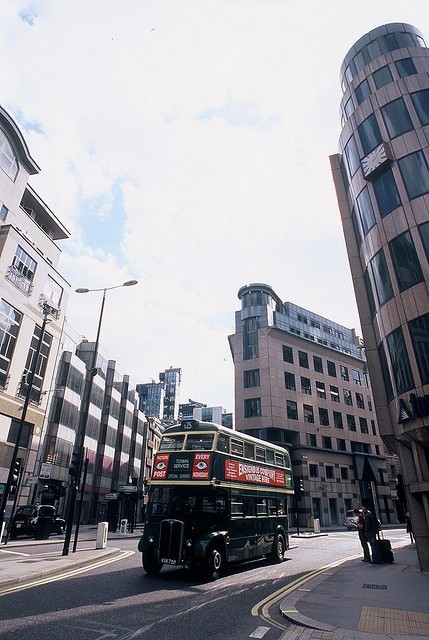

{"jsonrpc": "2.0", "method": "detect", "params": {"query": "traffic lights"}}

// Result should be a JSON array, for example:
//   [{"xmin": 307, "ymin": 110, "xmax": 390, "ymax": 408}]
[
  {"xmin": 295, "ymin": 476, "xmax": 304, "ymax": 494},
  {"xmin": 7, "ymin": 458, "xmax": 23, "ymax": 496},
  {"xmin": 68, "ymin": 449, "xmax": 80, "ymax": 480}
]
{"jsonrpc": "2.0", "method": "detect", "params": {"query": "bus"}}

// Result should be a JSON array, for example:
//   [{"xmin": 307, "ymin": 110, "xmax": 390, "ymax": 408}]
[{"xmin": 138, "ymin": 419, "xmax": 295, "ymax": 579}]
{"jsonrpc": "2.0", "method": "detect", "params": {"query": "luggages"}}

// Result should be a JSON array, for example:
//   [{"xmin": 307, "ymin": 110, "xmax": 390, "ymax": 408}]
[{"xmin": 375, "ymin": 531, "xmax": 394, "ymax": 564}]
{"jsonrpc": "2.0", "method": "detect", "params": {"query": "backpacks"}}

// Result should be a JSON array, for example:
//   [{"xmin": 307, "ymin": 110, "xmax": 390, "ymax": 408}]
[{"xmin": 372, "ymin": 516, "xmax": 382, "ymax": 531}]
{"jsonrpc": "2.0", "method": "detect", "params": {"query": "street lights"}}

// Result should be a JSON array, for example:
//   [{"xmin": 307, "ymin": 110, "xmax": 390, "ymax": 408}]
[{"xmin": 63, "ymin": 279, "xmax": 138, "ymax": 556}]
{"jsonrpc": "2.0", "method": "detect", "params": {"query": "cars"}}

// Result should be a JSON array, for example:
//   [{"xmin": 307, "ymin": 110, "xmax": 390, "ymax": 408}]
[{"xmin": 344, "ymin": 509, "xmax": 381, "ymax": 531}]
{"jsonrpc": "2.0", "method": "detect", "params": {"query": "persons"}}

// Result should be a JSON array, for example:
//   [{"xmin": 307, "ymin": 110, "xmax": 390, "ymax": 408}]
[
  {"xmin": 359, "ymin": 506, "xmax": 380, "ymax": 564},
  {"xmin": 352, "ymin": 509, "xmax": 371, "ymax": 562},
  {"xmin": 406, "ymin": 512, "xmax": 416, "ymax": 545}
]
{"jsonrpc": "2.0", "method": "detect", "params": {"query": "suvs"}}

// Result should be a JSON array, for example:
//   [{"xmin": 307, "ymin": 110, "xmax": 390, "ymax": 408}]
[{"xmin": 10, "ymin": 504, "xmax": 66, "ymax": 540}]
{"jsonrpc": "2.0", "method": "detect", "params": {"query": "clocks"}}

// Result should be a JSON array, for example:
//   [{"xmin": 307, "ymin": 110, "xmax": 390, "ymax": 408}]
[{"xmin": 359, "ymin": 141, "xmax": 395, "ymax": 182}]
